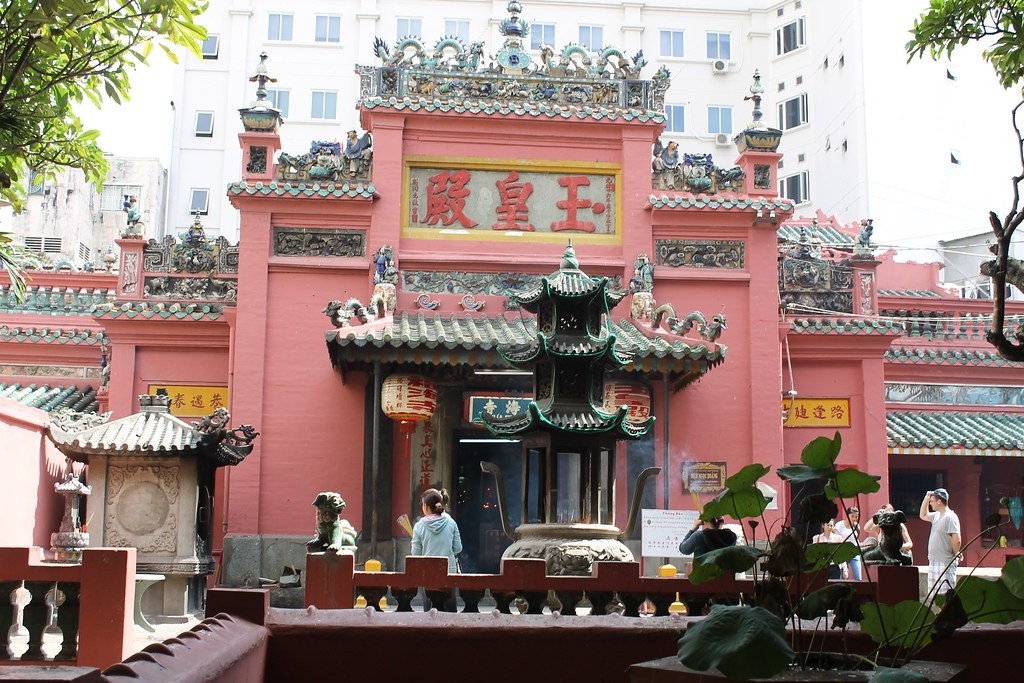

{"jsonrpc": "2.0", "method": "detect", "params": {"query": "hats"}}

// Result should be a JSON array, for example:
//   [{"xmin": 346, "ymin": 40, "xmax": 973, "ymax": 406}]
[{"xmin": 928, "ymin": 488, "xmax": 949, "ymax": 502}]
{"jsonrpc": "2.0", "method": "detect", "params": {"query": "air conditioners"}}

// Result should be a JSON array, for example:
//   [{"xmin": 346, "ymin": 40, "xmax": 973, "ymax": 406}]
[
  {"xmin": 712, "ymin": 59, "xmax": 728, "ymax": 74},
  {"xmin": 716, "ymin": 133, "xmax": 731, "ymax": 147}
]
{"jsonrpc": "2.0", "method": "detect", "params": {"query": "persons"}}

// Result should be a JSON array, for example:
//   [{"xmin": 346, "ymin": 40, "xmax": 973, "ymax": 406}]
[
  {"xmin": 919, "ymin": 488, "xmax": 963, "ymax": 600},
  {"xmin": 863, "ymin": 504, "xmax": 913, "ymax": 565},
  {"xmin": 813, "ymin": 519, "xmax": 848, "ymax": 579},
  {"xmin": 659, "ymin": 141, "xmax": 680, "ymax": 189},
  {"xmin": 836, "ymin": 506, "xmax": 862, "ymax": 581},
  {"xmin": 859, "ymin": 219, "xmax": 870, "ymax": 243},
  {"xmin": 373, "ymin": 244, "xmax": 398, "ymax": 284},
  {"xmin": 411, "ymin": 487, "xmax": 463, "ymax": 613},
  {"xmin": 680, "ymin": 513, "xmax": 739, "ymax": 617},
  {"xmin": 124, "ymin": 195, "xmax": 140, "ymax": 225},
  {"xmin": 630, "ymin": 258, "xmax": 655, "ymax": 291},
  {"xmin": 344, "ymin": 131, "xmax": 369, "ymax": 176}
]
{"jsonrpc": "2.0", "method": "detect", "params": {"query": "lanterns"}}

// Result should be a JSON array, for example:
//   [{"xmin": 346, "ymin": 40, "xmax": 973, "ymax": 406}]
[
  {"xmin": 382, "ymin": 374, "xmax": 437, "ymax": 457},
  {"xmin": 602, "ymin": 376, "xmax": 650, "ymax": 423}
]
